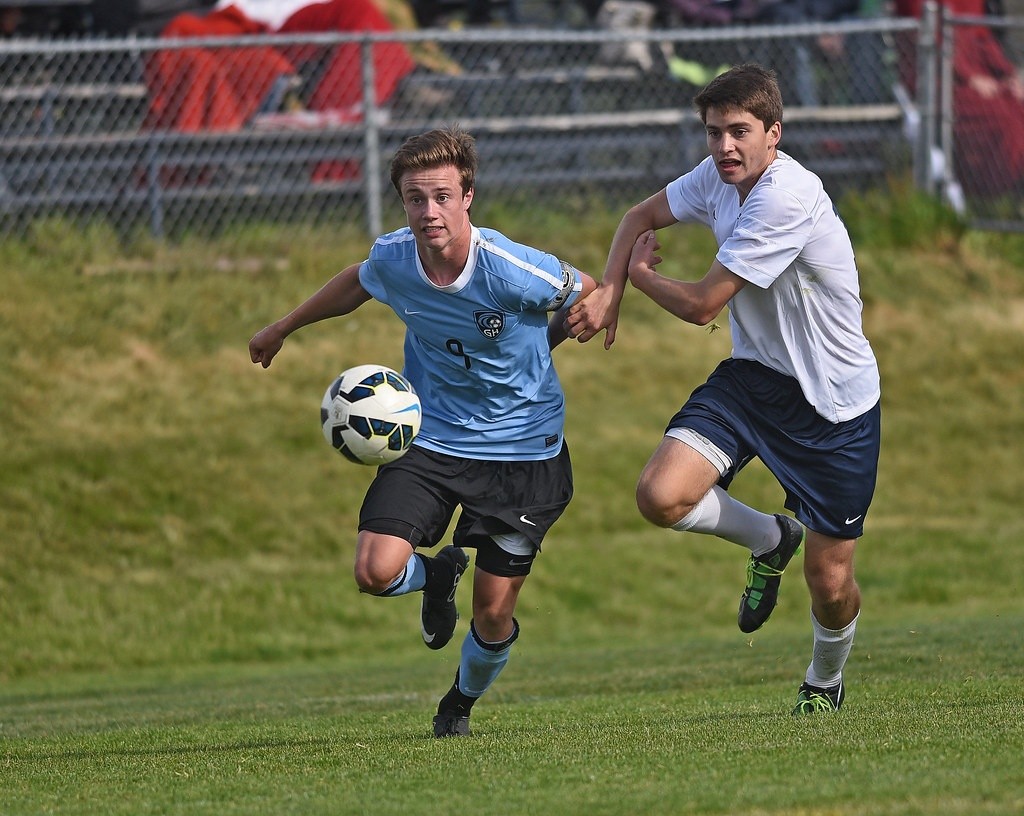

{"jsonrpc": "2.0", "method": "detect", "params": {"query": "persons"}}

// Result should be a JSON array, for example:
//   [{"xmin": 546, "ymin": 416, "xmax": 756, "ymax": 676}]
[
  {"xmin": 248, "ymin": 126, "xmax": 605, "ymax": 739},
  {"xmin": 567, "ymin": 64, "xmax": 883, "ymax": 721}
]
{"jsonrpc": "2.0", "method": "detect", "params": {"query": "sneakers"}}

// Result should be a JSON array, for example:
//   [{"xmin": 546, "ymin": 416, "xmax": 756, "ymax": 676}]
[
  {"xmin": 792, "ymin": 679, "xmax": 846, "ymax": 720},
  {"xmin": 736, "ymin": 512, "xmax": 804, "ymax": 633},
  {"xmin": 432, "ymin": 711, "xmax": 470, "ymax": 739},
  {"xmin": 421, "ymin": 545, "xmax": 469, "ymax": 650}
]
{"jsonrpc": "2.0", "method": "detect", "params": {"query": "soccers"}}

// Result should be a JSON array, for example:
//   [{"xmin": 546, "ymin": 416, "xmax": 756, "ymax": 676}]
[{"xmin": 321, "ymin": 364, "xmax": 422, "ymax": 467}]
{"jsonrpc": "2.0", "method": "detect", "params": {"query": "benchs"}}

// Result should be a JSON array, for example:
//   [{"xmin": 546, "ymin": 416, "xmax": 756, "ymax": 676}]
[{"xmin": 2, "ymin": 65, "xmax": 906, "ymax": 156}]
